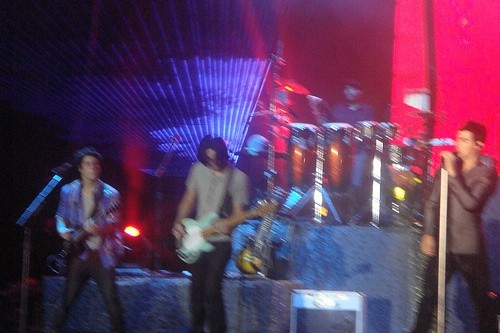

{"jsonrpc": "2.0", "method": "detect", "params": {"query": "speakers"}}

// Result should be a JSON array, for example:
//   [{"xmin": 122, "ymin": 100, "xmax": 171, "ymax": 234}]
[{"xmin": 289, "ymin": 289, "xmax": 368, "ymax": 333}]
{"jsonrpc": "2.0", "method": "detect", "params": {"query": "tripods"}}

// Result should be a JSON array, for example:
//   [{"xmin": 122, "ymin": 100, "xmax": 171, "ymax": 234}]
[{"xmin": 287, "ymin": 133, "xmax": 343, "ymax": 226}]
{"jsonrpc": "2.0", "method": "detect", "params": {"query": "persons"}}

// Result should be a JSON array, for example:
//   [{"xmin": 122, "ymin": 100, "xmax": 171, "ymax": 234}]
[
  {"xmin": 408, "ymin": 121, "xmax": 500, "ymax": 333},
  {"xmin": 170, "ymin": 133, "xmax": 250, "ymax": 333},
  {"xmin": 48, "ymin": 147, "xmax": 129, "ymax": 333},
  {"xmin": 329, "ymin": 80, "xmax": 377, "ymax": 229}
]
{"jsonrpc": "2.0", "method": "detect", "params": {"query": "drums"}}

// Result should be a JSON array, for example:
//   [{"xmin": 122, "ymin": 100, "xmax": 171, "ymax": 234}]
[
  {"xmin": 384, "ymin": 144, "xmax": 419, "ymax": 168},
  {"xmin": 321, "ymin": 121, "xmax": 355, "ymax": 145},
  {"xmin": 356, "ymin": 120, "xmax": 399, "ymax": 142},
  {"xmin": 287, "ymin": 123, "xmax": 320, "ymax": 147}
]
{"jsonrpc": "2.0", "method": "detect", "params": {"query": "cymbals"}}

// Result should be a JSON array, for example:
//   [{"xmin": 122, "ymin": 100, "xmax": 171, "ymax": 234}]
[{"xmin": 273, "ymin": 77, "xmax": 312, "ymax": 95}]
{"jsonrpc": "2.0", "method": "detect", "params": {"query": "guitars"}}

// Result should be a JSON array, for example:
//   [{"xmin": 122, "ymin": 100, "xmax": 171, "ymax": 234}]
[
  {"xmin": 46, "ymin": 197, "xmax": 121, "ymax": 274},
  {"xmin": 235, "ymin": 198, "xmax": 280, "ymax": 274},
  {"xmin": 172, "ymin": 197, "xmax": 281, "ymax": 264}
]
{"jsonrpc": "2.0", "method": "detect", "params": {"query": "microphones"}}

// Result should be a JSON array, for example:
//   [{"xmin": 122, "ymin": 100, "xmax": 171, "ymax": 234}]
[{"xmin": 51, "ymin": 162, "xmax": 73, "ymax": 174}]
